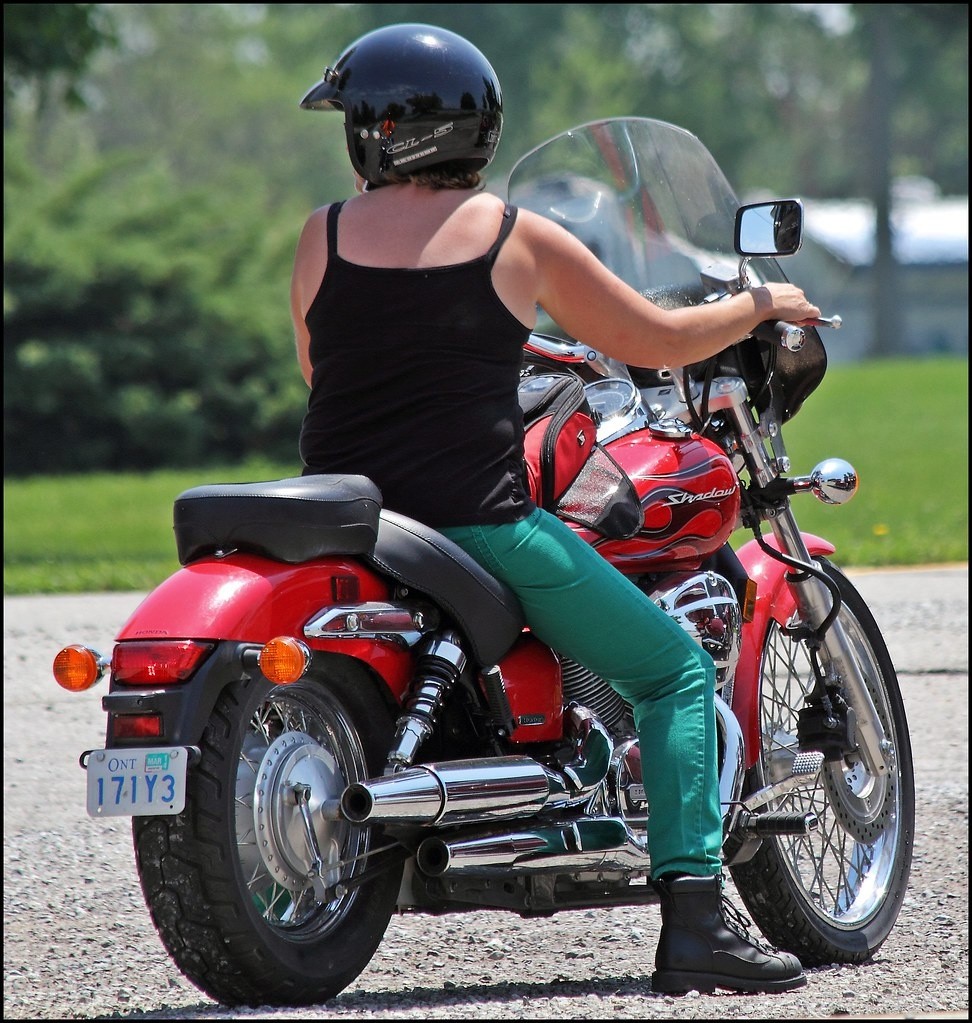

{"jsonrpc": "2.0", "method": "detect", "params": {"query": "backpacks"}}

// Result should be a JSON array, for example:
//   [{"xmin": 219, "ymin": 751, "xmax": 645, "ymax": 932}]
[{"xmin": 514, "ymin": 373, "xmax": 643, "ymax": 539}]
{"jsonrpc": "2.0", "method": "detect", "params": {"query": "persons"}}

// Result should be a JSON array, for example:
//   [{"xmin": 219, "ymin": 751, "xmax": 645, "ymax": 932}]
[{"xmin": 286, "ymin": 24, "xmax": 820, "ymax": 988}]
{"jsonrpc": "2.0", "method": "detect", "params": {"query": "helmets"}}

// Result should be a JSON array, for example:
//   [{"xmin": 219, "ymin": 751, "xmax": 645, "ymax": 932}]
[{"xmin": 299, "ymin": 23, "xmax": 501, "ymax": 190}]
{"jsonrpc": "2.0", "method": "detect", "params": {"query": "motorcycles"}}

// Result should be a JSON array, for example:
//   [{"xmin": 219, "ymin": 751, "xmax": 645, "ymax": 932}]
[{"xmin": 52, "ymin": 119, "xmax": 919, "ymax": 1009}]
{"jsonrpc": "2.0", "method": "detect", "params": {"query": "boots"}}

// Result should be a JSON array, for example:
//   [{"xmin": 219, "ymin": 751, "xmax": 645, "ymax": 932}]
[{"xmin": 648, "ymin": 875, "xmax": 806, "ymax": 995}]
